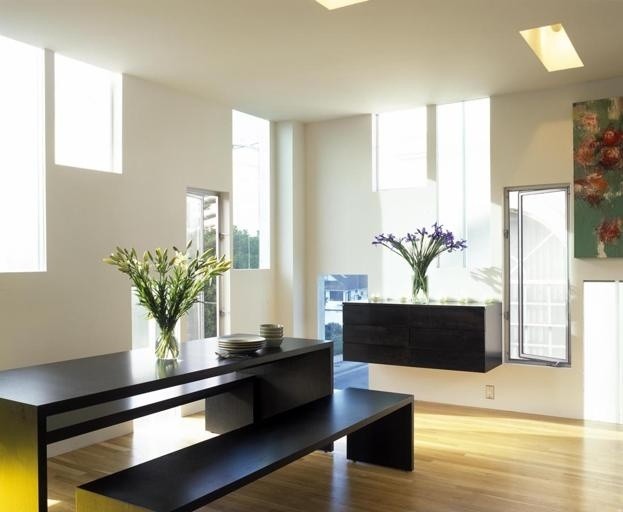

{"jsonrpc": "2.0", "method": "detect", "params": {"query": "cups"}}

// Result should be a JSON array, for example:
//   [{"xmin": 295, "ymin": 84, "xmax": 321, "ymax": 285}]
[{"xmin": 369, "ymin": 297, "xmax": 500, "ymax": 305}]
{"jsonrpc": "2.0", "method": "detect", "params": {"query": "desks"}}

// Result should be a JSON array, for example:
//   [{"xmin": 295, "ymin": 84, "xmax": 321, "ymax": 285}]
[{"xmin": 0, "ymin": 334, "xmax": 333, "ymax": 512}]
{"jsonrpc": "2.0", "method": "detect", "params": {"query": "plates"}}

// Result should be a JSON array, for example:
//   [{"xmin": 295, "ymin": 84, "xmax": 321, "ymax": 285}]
[{"xmin": 215, "ymin": 331, "xmax": 265, "ymax": 359}]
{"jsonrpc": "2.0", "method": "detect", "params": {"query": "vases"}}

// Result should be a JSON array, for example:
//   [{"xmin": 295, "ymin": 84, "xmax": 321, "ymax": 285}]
[{"xmin": 154, "ymin": 317, "xmax": 180, "ymax": 359}]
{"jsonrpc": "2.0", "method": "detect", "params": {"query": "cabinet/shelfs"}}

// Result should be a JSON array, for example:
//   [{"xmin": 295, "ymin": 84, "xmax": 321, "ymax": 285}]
[{"xmin": 343, "ymin": 303, "xmax": 502, "ymax": 373}]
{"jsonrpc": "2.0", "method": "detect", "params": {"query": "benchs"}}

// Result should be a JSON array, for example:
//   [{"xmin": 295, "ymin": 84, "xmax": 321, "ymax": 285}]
[
  {"xmin": 45, "ymin": 371, "xmax": 256, "ymax": 446},
  {"xmin": 75, "ymin": 387, "xmax": 414, "ymax": 512}
]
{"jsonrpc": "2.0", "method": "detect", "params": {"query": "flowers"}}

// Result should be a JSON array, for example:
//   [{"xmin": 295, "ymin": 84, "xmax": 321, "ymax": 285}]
[
  {"xmin": 102, "ymin": 240, "xmax": 232, "ymax": 360},
  {"xmin": 371, "ymin": 221, "xmax": 468, "ymax": 306}
]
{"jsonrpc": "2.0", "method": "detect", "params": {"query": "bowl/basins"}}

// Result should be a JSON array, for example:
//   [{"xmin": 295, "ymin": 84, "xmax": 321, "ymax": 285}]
[{"xmin": 260, "ymin": 323, "xmax": 284, "ymax": 349}]
